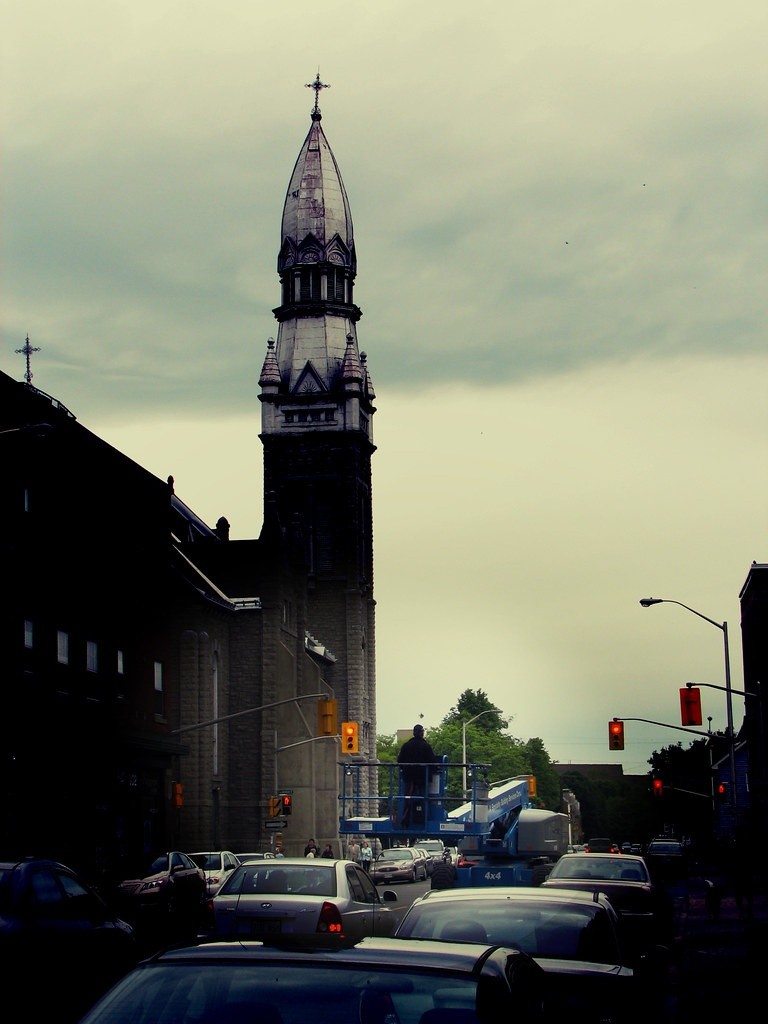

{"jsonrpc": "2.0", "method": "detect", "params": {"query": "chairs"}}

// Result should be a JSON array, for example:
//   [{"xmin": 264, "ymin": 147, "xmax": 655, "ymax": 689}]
[
  {"xmin": 532, "ymin": 916, "xmax": 589, "ymax": 957},
  {"xmin": 264, "ymin": 871, "xmax": 288, "ymax": 894},
  {"xmin": 419, "ymin": 1008, "xmax": 476, "ymax": 1024},
  {"xmin": 437, "ymin": 920, "xmax": 489, "ymax": 947},
  {"xmin": 622, "ymin": 868, "xmax": 642, "ymax": 882},
  {"xmin": 573, "ymin": 869, "xmax": 590, "ymax": 877}
]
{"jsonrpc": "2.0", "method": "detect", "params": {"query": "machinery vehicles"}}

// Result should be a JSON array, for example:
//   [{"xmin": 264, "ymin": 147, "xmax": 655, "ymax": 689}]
[{"xmin": 339, "ymin": 756, "xmax": 572, "ymax": 892}]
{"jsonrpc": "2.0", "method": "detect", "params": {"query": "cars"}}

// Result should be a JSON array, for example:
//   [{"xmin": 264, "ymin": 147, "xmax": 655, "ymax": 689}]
[
  {"xmin": 356, "ymin": 886, "xmax": 639, "ymax": 1024},
  {"xmin": 1, "ymin": 857, "xmax": 134, "ymax": 960},
  {"xmin": 541, "ymin": 850, "xmax": 658, "ymax": 945},
  {"xmin": 116, "ymin": 852, "xmax": 206, "ymax": 907},
  {"xmin": 78, "ymin": 942, "xmax": 568, "ymax": 1024},
  {"xmin": 571, "ymin": 839, "xmax": 685, "ymax": 871},
  {"xmin": 204, "ymin": 859, "xmax": 400, "ymax": 950},
  {"xmin": 187, "ymin": 851, "xmax": 242, "ymax": 894},
  {"xmin": 367, "ymin": 838, "xmax": 461, "ymax": 884},
  {"xmin": 236, "ymin": 854, "xmax": 282, "ymax": 880}
]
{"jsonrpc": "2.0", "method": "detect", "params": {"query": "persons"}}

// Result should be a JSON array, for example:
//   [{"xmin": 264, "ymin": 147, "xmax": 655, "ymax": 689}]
[
  {"xmin": 397, "ymin": 725, "xmax": 438, "ymax": 830},
  {"xmin": 362, "ymin": 841, "xmax": 372, "ymax": 873},
  {"xmin": 321, "ymin": 844, "xmax": 333, "ymax": 858},
  {"xmin": 348, "ymin": 839, "xmax": 360, "ymax": 863},
  {"xmin": 304, "ymin": 838, "xmax": 321, "ymax": 857}
]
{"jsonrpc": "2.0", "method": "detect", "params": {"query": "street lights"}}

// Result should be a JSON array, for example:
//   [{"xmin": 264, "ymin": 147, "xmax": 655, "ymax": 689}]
[
  {"xmin": 641, "ymin": 598, "xmax": 749, "ymax": 933},
  {"xmin": 462, "ymin": 708, "xmax": 501, "ymax": 809}
]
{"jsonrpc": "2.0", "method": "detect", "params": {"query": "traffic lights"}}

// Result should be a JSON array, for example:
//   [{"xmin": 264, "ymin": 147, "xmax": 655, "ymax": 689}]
[
  {"xmin": 282, "ymin": 796, "xmax": 291, "ymax": 817},
  {"xmin": 654, "ymin": 778, "xmax": 662, "ymax": 797},
  {"xmin": 342, "ymin": 722, "xmax": 358, "ymax": 754},
  {"xmin": 609, "ymin": 722, "xmax": 624, "ymax": 749},
  {"xmin": 716, "ymin": 786, "xmax": 727, "ymax": 802},
  {"xmin": 269, "ymin": 799, "xmax": 281, "ymax": 816}
]
